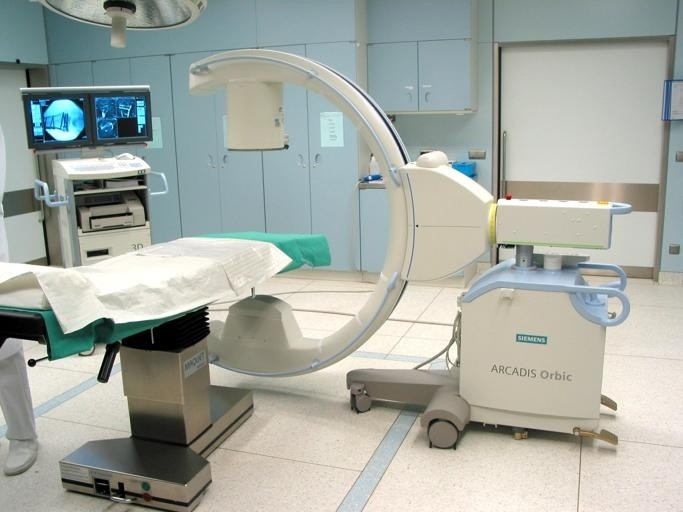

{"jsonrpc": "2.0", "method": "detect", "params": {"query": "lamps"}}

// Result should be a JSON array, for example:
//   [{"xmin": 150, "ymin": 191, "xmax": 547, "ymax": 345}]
[{"xmin": 39, "ymin": 0, "xmax": 208, "ymax": 49}]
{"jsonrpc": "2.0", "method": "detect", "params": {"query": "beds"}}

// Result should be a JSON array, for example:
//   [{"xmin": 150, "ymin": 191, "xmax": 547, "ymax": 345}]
[{"xmin": 0, "ymin": 231, "xmax": 329, "ymax": 511}]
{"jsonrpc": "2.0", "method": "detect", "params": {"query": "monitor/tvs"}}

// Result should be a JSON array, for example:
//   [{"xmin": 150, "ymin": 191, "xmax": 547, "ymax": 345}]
[
  {"xmin": 21, "ymin": 94, "xmax": 90, "ymax": 150},
  {"xmin": 89, "ymin": 92, "xmax": 153, "ymax": 144}
]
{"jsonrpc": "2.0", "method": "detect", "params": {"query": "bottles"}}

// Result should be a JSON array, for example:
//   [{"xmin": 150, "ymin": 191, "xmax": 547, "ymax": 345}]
[{"xmin": 369, "ymin": 156, "xmax": 380, "ymax": 175}]
